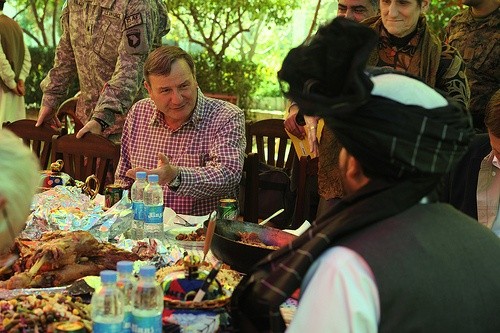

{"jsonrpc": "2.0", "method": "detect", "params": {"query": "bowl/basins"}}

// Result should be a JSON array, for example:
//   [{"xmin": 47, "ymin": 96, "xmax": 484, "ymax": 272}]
[{"xmin": 204, "ymin": 219, "xmax": 300, "ymax": 273}]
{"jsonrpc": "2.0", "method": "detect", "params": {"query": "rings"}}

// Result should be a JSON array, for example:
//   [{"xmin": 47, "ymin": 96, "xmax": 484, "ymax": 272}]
[{"xmin": 311, "ymin": 126, "xmax": 317, "ymax": 130}]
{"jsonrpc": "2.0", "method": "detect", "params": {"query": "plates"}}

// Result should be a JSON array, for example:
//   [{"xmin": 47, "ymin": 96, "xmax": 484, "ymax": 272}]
[{"xmin": 164, "ymin": 224, "xmax": 207, "ymax": 247}]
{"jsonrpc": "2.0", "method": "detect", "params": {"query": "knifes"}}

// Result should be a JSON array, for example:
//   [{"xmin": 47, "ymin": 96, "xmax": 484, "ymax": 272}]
[{"xmin": 194, "ymin": 260, "xmax": 224, "ymax": 300}]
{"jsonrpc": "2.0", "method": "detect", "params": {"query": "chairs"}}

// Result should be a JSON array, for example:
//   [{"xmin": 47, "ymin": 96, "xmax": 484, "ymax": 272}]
[
  {"xmin": 2, "ymin": 118, "xmax": 65, "ymax": 170},
  {"xmin": 239, "ymin": 119, "xmax": 317, "ymax": 231},
  {"xmin": 51, "ymin": 131, "xmax": 122, "ymax": 193}
]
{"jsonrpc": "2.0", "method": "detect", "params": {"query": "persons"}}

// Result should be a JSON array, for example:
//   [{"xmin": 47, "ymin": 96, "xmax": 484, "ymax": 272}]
[
  {"xmin": 434, "ymin": 0, "xmax": 500, "ymax": 130},
  {"xmin": 449, "ymin": 91, "xmax": 500, "ymax": 236},
  {"xmin": 338, "ymin": 0, "xmax": 379, "ymax": 23},
  {"xmin": 0, "ymin": 0, "xmax": 32, "ymax": 129},
  {"xmin": 226, "ymin": 15, "xmax": 500, "ymax": 333},
  {"xmin": 35, "ymin": 0, "xmax": 170, "ymax": 192},
  {"xmin": 0, "ymin": 125, "xmax": 40, "ymax": 257},
  {"xmin": 356, "ymin": 0, "xmax": 470, "ymax": 109},
  {"xmin": 115, "ymin": 46, "xmax": 246, "ymax": 217}
]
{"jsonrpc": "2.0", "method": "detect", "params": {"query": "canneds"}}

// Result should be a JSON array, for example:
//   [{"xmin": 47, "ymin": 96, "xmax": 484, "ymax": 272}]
[
  {"xmin": 217, "ymin": 198, "xmax": 237, "ymax": 221},
  {"xmin": 105, "ymin": 184, "xmax": 123, "ymax": 208}
]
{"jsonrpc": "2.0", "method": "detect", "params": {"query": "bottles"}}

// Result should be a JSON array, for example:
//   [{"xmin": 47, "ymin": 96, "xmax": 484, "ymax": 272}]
[
  {"xmin": 131, "ymin": 172, "xmax": 147, "ymax": 228},
  {"xmin": 116, "ymin": 260, "xmax": 136, "ymax": 333},
  {"xmin": 130, "ymin": 265, "xmax": 165, "ymax": 333},
  {"xmin": 143, "ymin": 175, "xmax": 165, "ymax": 234},
  {"xmin": 90, "ymin": 270, "xmax": 126, "ymax": 333}
]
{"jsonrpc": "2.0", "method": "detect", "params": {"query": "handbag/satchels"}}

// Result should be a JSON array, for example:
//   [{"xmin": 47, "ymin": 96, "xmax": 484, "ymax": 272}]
[{"xmin": 238, "ymin": 152, "xmax": 295, "ymax": 227}]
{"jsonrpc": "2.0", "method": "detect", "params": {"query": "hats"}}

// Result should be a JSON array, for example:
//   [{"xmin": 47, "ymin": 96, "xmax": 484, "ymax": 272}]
[{"xmin": 277, "ymin": 15, "xmax": 471, "ymax": 177}]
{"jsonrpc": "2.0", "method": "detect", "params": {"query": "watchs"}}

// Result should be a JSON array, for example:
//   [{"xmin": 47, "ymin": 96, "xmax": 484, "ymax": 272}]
[
  {"xmin": 166, "ymin": 171, "xmax": 182, "ymax": 189},
  {"xmin": 95, "ymin": 118, "xmax": 109, "ymax": 132}
]
